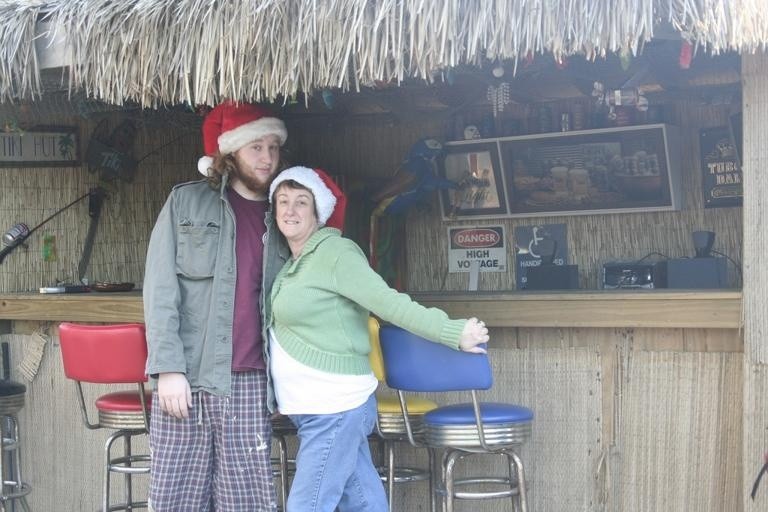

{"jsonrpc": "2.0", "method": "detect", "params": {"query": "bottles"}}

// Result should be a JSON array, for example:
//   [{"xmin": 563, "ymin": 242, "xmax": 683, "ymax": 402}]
[
  {"xmin": 447, "ymin": 173, "xmax": 473, "ymax": 219},
  {"xmin": 526, "ymin": 100, "xmax": 551, "ymax": 134},
  {"xmin": 571, "ymin": 98, "xmax": 585, "ymax": 129}
]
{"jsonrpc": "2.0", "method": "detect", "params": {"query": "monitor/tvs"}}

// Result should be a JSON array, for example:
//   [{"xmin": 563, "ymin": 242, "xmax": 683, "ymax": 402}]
[{"xmin": 528, "ymin": 264, "xmax": 578, "ymax": 290}]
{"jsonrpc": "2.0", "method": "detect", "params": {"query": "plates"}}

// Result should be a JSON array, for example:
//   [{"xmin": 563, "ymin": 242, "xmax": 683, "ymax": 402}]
[
  {"xmin": 523, "ymin": 198, "xmax": 568, "ymax": 207},
  {"xmin": 91, "ymin": 282, "xmax": 136, "ymax": 292}
]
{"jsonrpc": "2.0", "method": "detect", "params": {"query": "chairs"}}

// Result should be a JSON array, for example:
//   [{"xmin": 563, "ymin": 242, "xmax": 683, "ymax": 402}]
[
  {"xmin": 348, "ymin": 312, "xmax": 438, "ymax": 512},
  {"xmin": 56, "ymin": 322, "xmax": 158, "ymax": 512},
  {"xmin": 0, "ymin": 378, "xmax": 35, "ymax": 512},
  {"xmin": 375, "ymin": 320, "xmax": 535, "ymax": 512},
  {"xmin": 260, "ymin": 412, "xmax": 298, "ymax": 512}
]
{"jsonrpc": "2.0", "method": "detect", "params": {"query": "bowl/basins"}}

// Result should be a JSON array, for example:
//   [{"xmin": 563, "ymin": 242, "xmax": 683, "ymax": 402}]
[{"xmin": 613, "ymin": 172, "xmax": 661, "ymax": 202}]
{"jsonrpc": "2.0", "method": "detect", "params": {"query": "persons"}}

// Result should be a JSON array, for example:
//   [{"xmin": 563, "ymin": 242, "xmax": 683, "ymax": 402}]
[
  {"xmin": 143, "ymin": 98, "xmax": 287, "ymax": 511},
  {"xmin": 261, "ymin": 165, "xmax": 490, "ymax": 511}
]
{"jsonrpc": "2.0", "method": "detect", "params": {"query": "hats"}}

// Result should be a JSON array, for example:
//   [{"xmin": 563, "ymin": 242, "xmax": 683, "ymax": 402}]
[
  {"xmin": 268, "ymin": 164, "xmax": 348, "ymax": 234},
  {"xmin": 197, "ymin": 100, "xmax": 289, "ymax": 178}
]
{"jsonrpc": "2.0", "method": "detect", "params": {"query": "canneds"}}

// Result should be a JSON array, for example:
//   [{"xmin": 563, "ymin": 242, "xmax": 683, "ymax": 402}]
[{"xmin": 2, "ymin": 223, "xmax": 29, "ymax": 247}]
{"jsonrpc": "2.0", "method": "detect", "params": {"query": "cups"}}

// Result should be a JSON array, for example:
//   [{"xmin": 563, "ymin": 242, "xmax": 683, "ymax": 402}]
[
  {"xmin": 622, "ymin": 154, "xmax": 659, "ymax": 176},
  {"xmin": 552, "ymin": 169, "xmax": 568, "ymax": 194},
  {"xmin": 501, "ymin": 117, "xmax": 520, "ymax": 135},
  {"xmin": 560, "ymin": 112, "xmax": 570, "ymax": 132},
  {"xmin": 691, "ymin": 230, "xmax": 717, "ymax": 257},
  {"xmin": 647, "ymin": 104, "xmax": 664, "ymax": 124},
  {"xmin": 569, "ymin": 169, "xmax": 590, "ymax": 200},
  {"xmin": 615, "ymin": 104, "xmax": 637, "ymax": 126}
]
